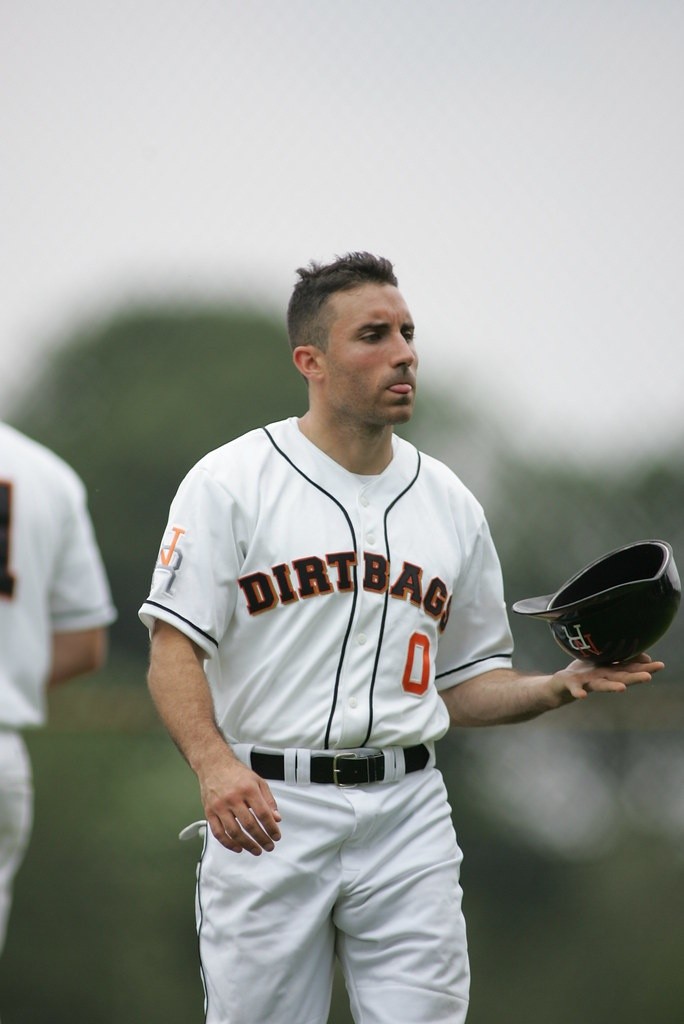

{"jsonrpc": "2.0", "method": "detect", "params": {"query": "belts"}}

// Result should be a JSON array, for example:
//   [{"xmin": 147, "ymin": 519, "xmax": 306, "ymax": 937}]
[{"xmin": 250, "ymin": 744, "xmax": 430, "ymax": 788}]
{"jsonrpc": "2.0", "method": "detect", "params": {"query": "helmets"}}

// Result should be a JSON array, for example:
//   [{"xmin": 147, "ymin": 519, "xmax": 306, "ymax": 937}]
[{"xmin": 513, "ymin": 539, "xmax": 682, "ymax": 667}]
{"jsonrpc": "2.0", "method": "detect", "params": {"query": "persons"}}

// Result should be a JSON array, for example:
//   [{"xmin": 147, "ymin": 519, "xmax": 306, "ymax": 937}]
[
  {"xmin": 0, "ymin": 418, "xmax": 116, "ymax": 1024},
  {"xmin": 142, "ymin": 250, "xmax": 666, "ymax": 1024}
]
